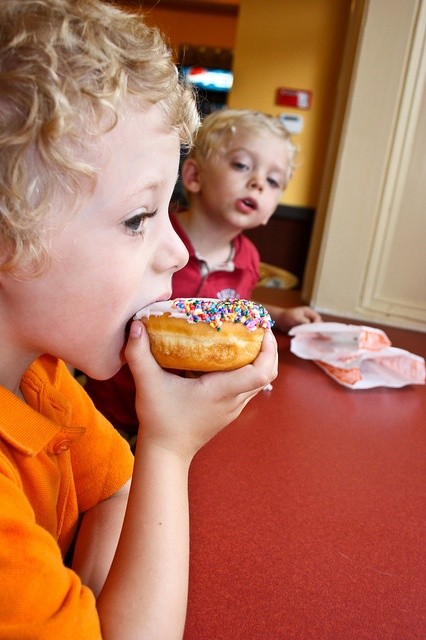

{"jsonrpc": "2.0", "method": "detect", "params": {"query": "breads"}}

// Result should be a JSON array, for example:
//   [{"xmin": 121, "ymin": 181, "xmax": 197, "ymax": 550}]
[{"xmin": 132, "ymin": 294, "xmax": 273, "ymax": 375}]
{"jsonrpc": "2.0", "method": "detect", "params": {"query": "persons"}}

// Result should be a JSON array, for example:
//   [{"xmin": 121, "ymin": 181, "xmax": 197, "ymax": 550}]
[
  {"xmin": 0, "ymin": 0, "xmax": 282, "ymax": 640},
  {"xmin": 85, "ymin": 109, "xmax": 323, "ymax": 438}
]
